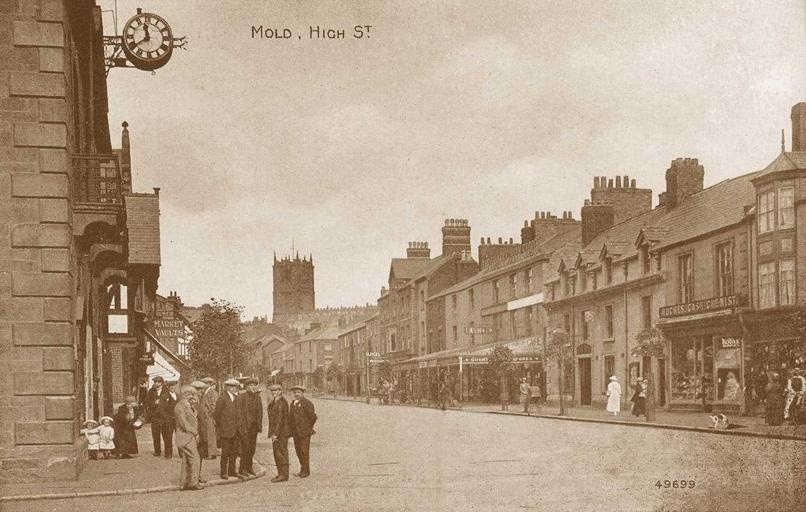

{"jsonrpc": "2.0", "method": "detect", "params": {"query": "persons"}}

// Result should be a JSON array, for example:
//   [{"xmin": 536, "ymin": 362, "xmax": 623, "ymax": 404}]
[
  {"xmin": 519, "ymin": 377, "xmax": 530, "ymax": 413},
  {"xmin": 267, "ymin": 384, "xmax": 291, "ymax": 483},
  {"xmin": 88, "ymin": 416, "xmax": 115, "ymax": 459},
  {"xmin": 765, "ymin": 373, "xmax": 784, "ymax": 426},
  {"xmin": 631, "ymin": 377, "xmax": 647, "ymax": 417},
  {"xmin": 289, "ymin": 385, "xmax": 317, "ymax": 478},
  {"xmin": 784, "ymin": 368, "xmax": 806, "ymax": 426},
  {"xmin": 606, "ymin": 376, "xmax": 621, "ymax": 416},
  {"xmin": 147, "ymin": 376, "xmax": 263, "ymax": 490},
  {"xmin": 79, "ymin": 420, "xmax": 99, "ymax": 460},
  {"xmin": 114, "ymin": 396, "xmax": 144, "ymax": 459}
]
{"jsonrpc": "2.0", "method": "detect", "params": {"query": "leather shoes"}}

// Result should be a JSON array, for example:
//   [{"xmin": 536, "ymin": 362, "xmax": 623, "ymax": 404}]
[
  {"xmin": 296, "ymin": 471, "xmax": 310, "ymax": 478},
  {"xmin": 220, "ymin": 470, "xmax": 256, "ymax": 480},
  {"xmin": 182, "ymin": 476, "xmax": 207, "ymax": 491},
  {"xmin": 271, "ymin": 475, "xmax": 288, "ymax": 482}
]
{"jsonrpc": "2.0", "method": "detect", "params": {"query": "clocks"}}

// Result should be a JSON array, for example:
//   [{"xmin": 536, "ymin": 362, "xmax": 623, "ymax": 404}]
[{"xmin": 121, "ymin": 11, "xmax": 174, "ymax": 70}]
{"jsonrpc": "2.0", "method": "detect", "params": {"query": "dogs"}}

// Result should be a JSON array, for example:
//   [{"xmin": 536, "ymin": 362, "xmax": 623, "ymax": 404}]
[{"xmin": 709, "ymin": 414, "xmax": 730, "ymax": 430}]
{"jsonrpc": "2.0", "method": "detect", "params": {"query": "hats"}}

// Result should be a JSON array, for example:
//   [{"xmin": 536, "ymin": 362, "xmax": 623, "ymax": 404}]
[
  {"xmin": 151, "ymin": 375, "xmax": 164, "ymax": 381},
  {"xmin": 222, "ymin": 378, "xmax": 240, "ymax": 387},
  {"xmin": 165, "ymin": 378, "xmax": 178, "ymax": 385},
  {"xmin": 609, "ymin": 376, "xmax": 617, "ymax": 380},
  {"xmin": 289, "ymin": 386, "xmax": 307, "ymax": 391},
  {"xmin": 82, "ymin": 419, "xmax": 99, "ymax": 426},
  {"xmin": 181, "ymin": 376, "xmax": 214, "ymax": 394},
  {"xmin": 245, "ymin": 377, "xmax": 259, "ymax": 385},
  {"xmin": 99, "ymin": 415, "xmax": 114, "ymax": 424},
  {"xmin": 269, "ymin": 385, "xmax": 284, "ymax": 392}
]
